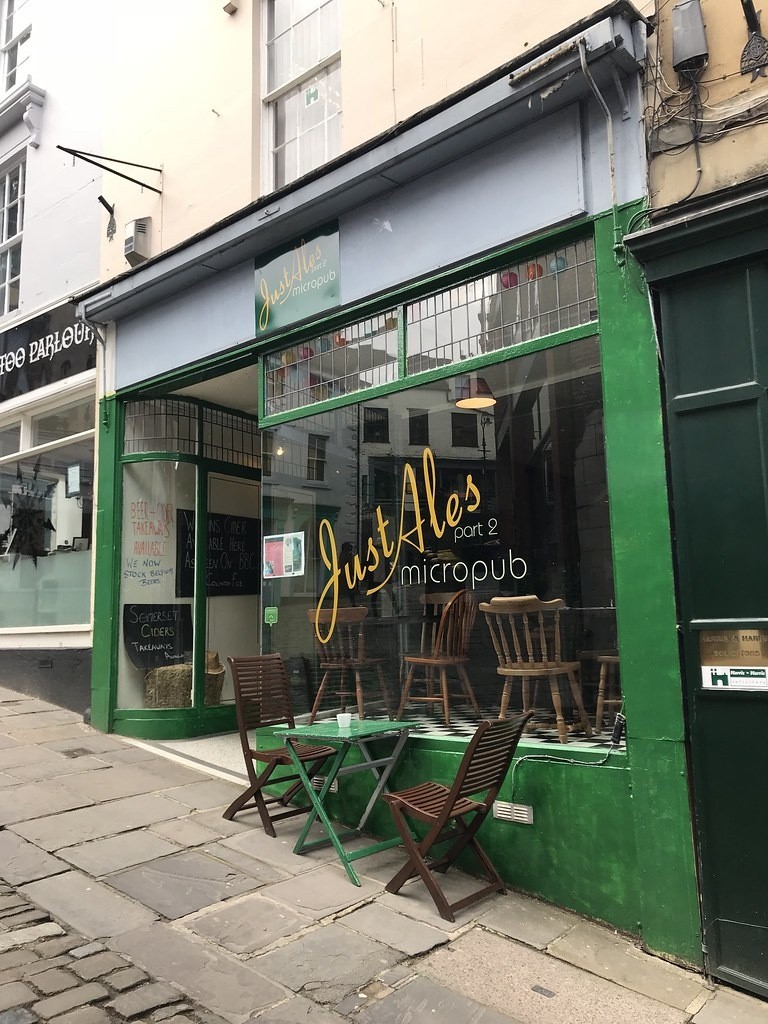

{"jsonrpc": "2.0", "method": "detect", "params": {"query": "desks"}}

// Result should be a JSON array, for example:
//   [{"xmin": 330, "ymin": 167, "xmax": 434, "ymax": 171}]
[
  {"xmin": 343, "ymin": 615, "xmax": 440, "ymax": 714},
  {"xmin": 274, "ymin": 720, "xmax": 423, "ymax": 887},
  {"xmin": 526, "ymin": 607, "xmax": 616, "ymax": 723}
]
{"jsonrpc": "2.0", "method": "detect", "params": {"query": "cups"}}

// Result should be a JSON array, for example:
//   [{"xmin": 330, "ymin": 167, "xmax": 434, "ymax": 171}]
[{"xmin": 336, "ymin": 713, "xmax": 351, "ymax": 728}]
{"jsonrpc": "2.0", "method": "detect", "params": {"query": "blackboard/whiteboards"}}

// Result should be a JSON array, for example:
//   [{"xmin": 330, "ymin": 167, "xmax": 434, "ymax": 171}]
[
  {"xmin": 176, "ymin": 508, "xmax": 261, "ymax": 597},
  {"xmin": 123, "ymin": 604, "xmax": 193, "ymax": 671}
]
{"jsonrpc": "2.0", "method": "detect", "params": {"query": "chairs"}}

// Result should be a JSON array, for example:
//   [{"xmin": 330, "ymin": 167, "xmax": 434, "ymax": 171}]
[
  {"xmin": 595, "ymin": 655, "xmax": 623, "ymax": 733},
  {"xmin": 222, "ymin": 653, "xmax": 338, "ymax": 838},
  {"xmin": 382, "ymin": 709, "xmax": 535, "ymax": 918},
  {"xmin": 395, "ymin": 589, "xmax": 478, "ymax": 724},
  {"xmin": 479, "ymin": 595, "xmax": 593, "ymax": 744},
  {"xmin": 306, "ymin": 607, "xmax": 394, "ymax": 726}
]
